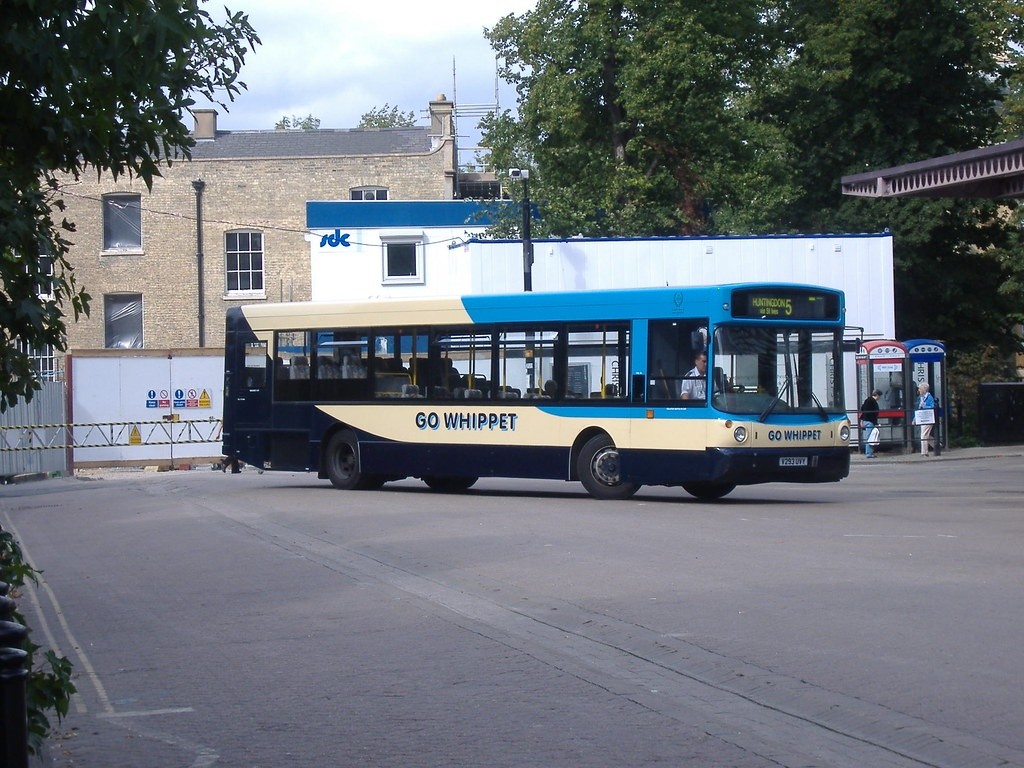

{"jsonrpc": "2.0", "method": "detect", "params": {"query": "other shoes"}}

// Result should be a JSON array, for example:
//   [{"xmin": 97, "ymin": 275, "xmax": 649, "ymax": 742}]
[
  {"xmin": 866, "ymin": 455, "xmax": 877, "ymax": 458},
  {"xmin": 921, "ymin": 454, "xmax": 928, "ymax": 457},
  {"xmin": 941, "ymin": 444, "xmax": 944, "ymax": 450}
]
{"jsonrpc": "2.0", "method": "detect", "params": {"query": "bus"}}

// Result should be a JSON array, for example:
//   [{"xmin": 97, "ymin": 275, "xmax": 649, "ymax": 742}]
[{"xmin": 221, "ymin": 281, "xmax": 865, "ymax": 502}]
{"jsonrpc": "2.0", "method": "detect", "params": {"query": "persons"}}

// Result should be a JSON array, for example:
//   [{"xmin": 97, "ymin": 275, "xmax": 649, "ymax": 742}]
[
  {"xmin": 858, "ymin": 389, "xmax": 884, "ymax": 458},
  {"xmin": 681, "ymin": 352, "xmax": 721, "ymax": 400},
  {"xmin": 913, "ymin": 381, "xmax": 944, "ymax": 457}
]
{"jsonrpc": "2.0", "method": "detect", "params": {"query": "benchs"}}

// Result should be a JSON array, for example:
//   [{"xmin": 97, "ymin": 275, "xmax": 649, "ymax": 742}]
[
  {"xmin": 409, "ymin": 358, "xmax": 460, "ymax": 387},
  {"xmin": 448, "ymin": 374, "xmax": 487, "ymax": 386}
]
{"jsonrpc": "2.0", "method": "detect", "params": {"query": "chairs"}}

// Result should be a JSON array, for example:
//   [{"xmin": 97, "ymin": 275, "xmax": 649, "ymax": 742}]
[
  {"xmin": 318, "ymin": 355, "xmax": 342, "ymax": 379},
  {"xmin": 341, "ymin": 355, "xmax": 367, "ymax": 378},
  {"xmin": 527, "ymin": 388, "xmax": 544, "ymax": 395},
  {"xmin": 464, "ymin": 389, "xmax": 483, "ymax": 399},
  {"xmin": 289, "ymin": 356, "xmax": 310, "ymax": 379},
  {"xmin": 499, "ymin": 392, "xmax": 518, "ymax": 399},
  {"xmin": 276, "ymin": 357, "xmax": 289, "ymax": 379},
  {"xmin": 401, "ymin": 384, "xmax": 424, "ymax": 398}
]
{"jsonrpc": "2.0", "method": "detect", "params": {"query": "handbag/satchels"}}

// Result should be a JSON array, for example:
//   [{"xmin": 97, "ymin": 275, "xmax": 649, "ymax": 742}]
[
  {"xmin": 912, "ymin": 418, "xmax": 916, "ymax": 426},
  {"xmin": 867, "ymin": 424, "xmax": 881, "ymax": 445}
]
